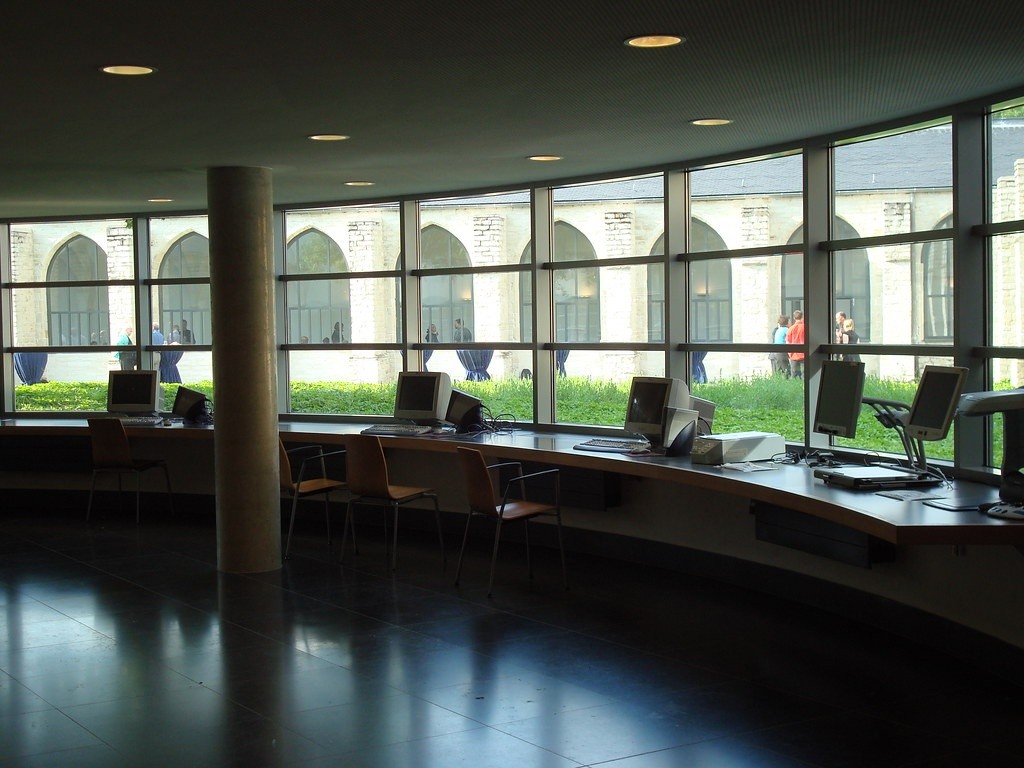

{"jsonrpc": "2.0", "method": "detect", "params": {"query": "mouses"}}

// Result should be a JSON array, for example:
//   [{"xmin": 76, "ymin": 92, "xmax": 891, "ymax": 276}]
[
  {"xmin": 433, "ymin": 430, "xmax": 449, "ymax": 435},
  {"xmin": 632, "ymin": 448, "xmax": 651, "ymax": 454},
  {"xmin": 164, "ymin": 421, "xmax": 172, "ymax": 426}
]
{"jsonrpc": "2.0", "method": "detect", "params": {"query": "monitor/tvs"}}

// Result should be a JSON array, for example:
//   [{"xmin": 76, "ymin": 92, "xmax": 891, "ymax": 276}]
[
  {"xmin": 625, "ymin": 377, "xmax": 716, "ymax": 448},
  {"xmin": 813, "ymin": 360, "xmax": 865, "ymax": 438},
  {"xmin": 905, "ymin": 365, "xmax": 969, "ymax": 441},
  {"xmin": 395, "ymin": 372, "xmax": 451, "ymax": 427},
  {"xmin": 107, "ymin": 370, "xmax": 159, "ymax": 419}
]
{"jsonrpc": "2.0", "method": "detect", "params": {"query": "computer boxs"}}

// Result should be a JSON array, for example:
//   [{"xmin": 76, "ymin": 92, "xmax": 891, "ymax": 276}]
[
  {"xmin": 661, "ymin": 407, "xmax": 699, "ymax": 448},
  {"xmin": 445, "ymin": 389, "xmax": 482, "ymax": 429},
  {"xmin": 173, "ymin": 386, "xmax": 206, "ymax": 422}
]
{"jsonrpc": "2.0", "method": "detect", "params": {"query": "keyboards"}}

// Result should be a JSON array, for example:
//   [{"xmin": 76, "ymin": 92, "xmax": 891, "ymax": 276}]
[
  {"xmin": 109, "ymin": 416, "xmax": 163, "ymax": 426},
  {"xmin": 361, "ymin": 424, "xmax": 431, "ymax": 437},
  {"xmin": 573, "ymin": 438, "xmax": 651, "ymax": 453}
]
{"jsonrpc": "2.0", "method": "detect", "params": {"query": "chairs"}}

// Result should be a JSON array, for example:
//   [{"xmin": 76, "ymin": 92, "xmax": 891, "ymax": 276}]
[
  {"xmin": 455, "ymin": 445, "xmax": 564, "ymax": 598},
  {"xmin": 278, "ymin": 438, "xmax": 357, "ymax": 561},
  {"xmin": 338, "ymin": 431, "xmax": 447, "ymax": 577},
  {"xmin": 84, "ymin": 417, "xmax": 173, "ymax": 526}
]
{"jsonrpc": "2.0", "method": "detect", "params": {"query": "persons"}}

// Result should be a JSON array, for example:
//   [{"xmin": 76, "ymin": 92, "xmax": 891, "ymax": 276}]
[
  {"xmin": 332, "ymin": 322, "xmax": 348, "ymax": 342},
  {"xmin": 455, "ymin": 318, "xmax": 472, "ymax": 341},
  {"xmin": 425, "ymin": 324, "xmax": 439, "ymax": 342},
  {"xmin": 91, "ymin": 341, "xmax": 98, "ymax": 346},
  {"xmin": 152, "ymin": 322, "xmax": 164, "ymax": 345},
  {"xmin": 167, "ymin": 324, "xmax": 181, "ymax": 344},
  {"xmin": 179, "ymin": 320, "xmax": 196, "ymax": 344},
  {"xmin": 767, "ymin": 310, "xmax": 804, "ymax": 378},
  {"xmin": 300, "ymin": 336, "xmax": 309, "ymax": 344},
  {"xmin": 322, "ymin": 337, "xmax": 329, "ymax": 343},
  {"xmin": 113, "ymin": 325, "xmax": 136, "ymax": 367},
  {"xmin": 835, "ymin": 311, "xmax": 861, "ymax": 362}
]
